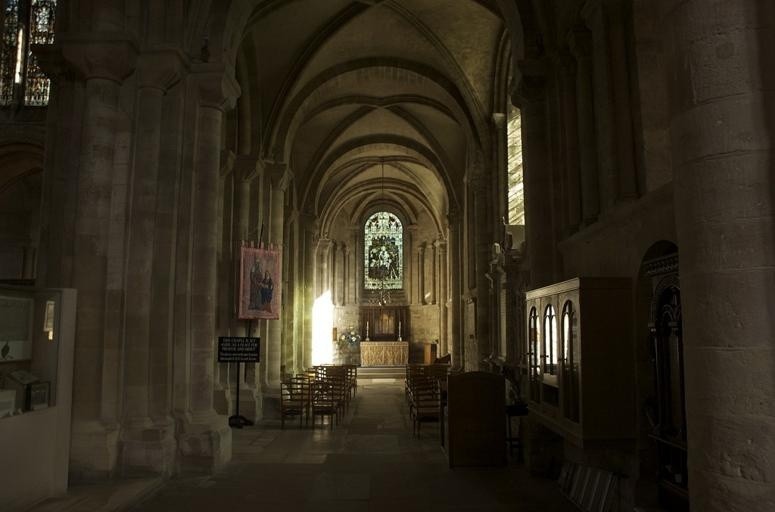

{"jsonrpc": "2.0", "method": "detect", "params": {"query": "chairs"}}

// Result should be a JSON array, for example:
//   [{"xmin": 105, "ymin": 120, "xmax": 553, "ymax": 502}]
[
  {"xmin": 406, "ymin": 363, "xmax": 512, "ymax": 470},
  {"xmin": 282, "ymin": 365, "xmax": 357, "ymax": 430}
]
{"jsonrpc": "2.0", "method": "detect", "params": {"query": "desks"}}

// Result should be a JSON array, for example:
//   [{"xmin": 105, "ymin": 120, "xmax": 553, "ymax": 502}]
[{"xmin": 360, "ymin": 340, "xmax": 409, "ymax": 368}]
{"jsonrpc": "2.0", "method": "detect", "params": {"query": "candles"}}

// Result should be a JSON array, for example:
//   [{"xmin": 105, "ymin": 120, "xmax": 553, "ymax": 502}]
[
  {"xmin": 367, "ymin": 321, "xmax": 368, "ymax": 327},
  {"xmin": 399, "ymin": 321, "xmax": 401, "ymax": 328}
]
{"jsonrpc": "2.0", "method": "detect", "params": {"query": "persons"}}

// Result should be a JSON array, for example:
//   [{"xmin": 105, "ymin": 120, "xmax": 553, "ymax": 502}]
[
  {"xmin": 248, "ymin": 259, "xmax": 263, "ymax": 308},
  {"xmin": 262, "ymin": 270, "xmax": 274, "ymax": 313}
]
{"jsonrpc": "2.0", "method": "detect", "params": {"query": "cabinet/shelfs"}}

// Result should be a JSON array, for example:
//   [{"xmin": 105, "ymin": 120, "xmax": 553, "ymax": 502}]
[{"xmin": 525, "ymin": 276, "xmax": 633, "ymax": 450}]
